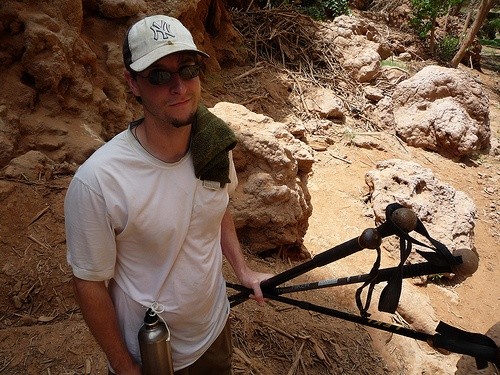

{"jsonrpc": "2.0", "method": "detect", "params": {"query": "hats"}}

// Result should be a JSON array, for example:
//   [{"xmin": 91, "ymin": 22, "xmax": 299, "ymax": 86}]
[{"xmin": 122, "ymin": 15, "xmax": 210, "ymax": 73}]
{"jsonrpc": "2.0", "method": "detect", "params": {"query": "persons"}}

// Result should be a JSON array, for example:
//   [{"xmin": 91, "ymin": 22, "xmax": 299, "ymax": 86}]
[{"xmin": 63, "ymin": 15, "xmax": 279, "ymax": 375}]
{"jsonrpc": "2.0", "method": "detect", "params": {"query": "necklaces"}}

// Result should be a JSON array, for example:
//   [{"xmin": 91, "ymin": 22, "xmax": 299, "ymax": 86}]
[{"xmin": 135, "ymin": 125, "xmax": 190, "ymax": 163}]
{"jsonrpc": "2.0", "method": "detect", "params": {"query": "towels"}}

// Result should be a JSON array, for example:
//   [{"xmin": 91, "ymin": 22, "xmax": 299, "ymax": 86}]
[{"xmin": 189, "ymin": 103, "xmax": 238, "ymax": 190}]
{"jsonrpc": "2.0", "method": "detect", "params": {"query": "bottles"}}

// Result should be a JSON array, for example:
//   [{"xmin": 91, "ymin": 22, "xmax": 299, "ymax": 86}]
[{"xmin": 137, "ymin": 308, "xmax": 174, "ymax": 375}]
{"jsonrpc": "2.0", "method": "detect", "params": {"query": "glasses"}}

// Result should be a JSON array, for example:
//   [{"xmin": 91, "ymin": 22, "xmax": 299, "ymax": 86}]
[{"xmin": 135, "ymin": 62, "xmax": 204, "ymax": 85}]
